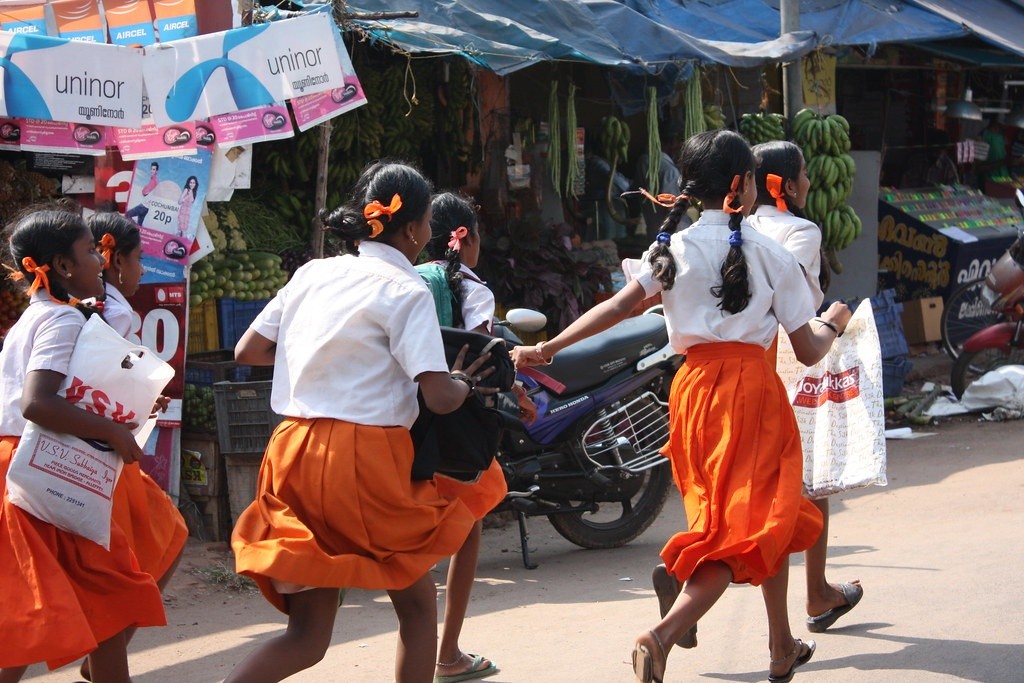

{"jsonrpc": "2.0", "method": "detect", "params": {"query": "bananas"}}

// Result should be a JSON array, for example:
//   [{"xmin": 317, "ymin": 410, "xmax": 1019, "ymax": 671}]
[
  {"xmin": 705, "ymin": 103, "xmax": 863, "ymax": 274},
  {"xmin": 600, "ymin": 115, "xmax": 631, "ymax": 163},
  {"xmin": 267, "ymin": 59, "xmax": 477, "ymax": 238}
]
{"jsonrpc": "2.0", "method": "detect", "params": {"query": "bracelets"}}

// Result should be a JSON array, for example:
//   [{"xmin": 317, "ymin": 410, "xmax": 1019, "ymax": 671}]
[
  {"xmin": 517, "ymin": 387, "xmax": 527, "ymax": 400},
  {"xmin": 535, "ymin": 341, "xmax": 553, "ymax": 367},
  {"xmin": 820, "ymin": 321, "xmax": 840, "ymax": 337},
  {"xmin": 450, "ymin": 373, "xmax": 474, "ymax": 394}
]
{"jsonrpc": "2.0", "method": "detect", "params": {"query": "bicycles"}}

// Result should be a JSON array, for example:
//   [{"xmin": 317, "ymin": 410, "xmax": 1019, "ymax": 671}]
[{"xmin": 940, "ymin": 275, "xmax": 1024, "ymax": 375}]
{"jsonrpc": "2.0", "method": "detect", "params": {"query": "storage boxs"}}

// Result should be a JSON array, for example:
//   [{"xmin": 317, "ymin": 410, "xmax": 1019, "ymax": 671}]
[
  {"xmin": 181, "ymin": 298, "xmax": 286, "ymax": 543},
  {"xmin": 984, "ymin": 180, "xmax": 1022, "ymax": 198},
  {"xmin": 495, "ymin": 303, "xmax": 546, "ymax": 346},
  {"xmin": 595, "ymin": 290, "xmax": 665, "ymax": 319},
  {"xmin": 847, "ymin": 289, "xmax": 944, "ymax": 397}
]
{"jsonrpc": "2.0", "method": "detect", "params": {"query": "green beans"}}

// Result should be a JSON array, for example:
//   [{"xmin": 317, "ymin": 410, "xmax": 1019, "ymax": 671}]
[
  {"xmin": 548, "ymin": 80, "xmax": 580, "ymax": 200},
  {"xmin": 683, "ymin": 63, "xmax": 707, "ymax": 141},
  {"xmin": 227, "ymin": 192, "xmax": 308, "ymax": 253},
  {"xmin": 646, "ymin": 86, "xmax": 662, "ymax": 213}
]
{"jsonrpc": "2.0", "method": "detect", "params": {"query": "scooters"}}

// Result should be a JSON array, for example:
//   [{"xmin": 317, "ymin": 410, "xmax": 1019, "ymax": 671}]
[
  {"xmin": 491, "ymin": 303, "xmax": 688, "ymax": 570},
  {"xmin": 949, "ymin": 247, "xmax": 1024, "ymax": 401}
]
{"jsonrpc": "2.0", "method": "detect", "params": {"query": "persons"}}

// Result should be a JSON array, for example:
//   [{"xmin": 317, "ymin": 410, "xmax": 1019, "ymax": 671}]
[
  {"xmin": 224, "ymin": 160, "xmax": 499, "ymax": 683},
  {"xmin": 624, "ymin": 139, "xmax": 862, "ymax": 647},
  {"xmin": 633, "ymin": 118, "xmax": 685, "ymax": 242},
  {"xmin": 127, "ymin": 162, "xmax": 159, "ymax": 226},
  {"xmin": 505, "ymin": 128, "xmax": 849, "ymax": 683},
  {"xmin": 974, "ymin": 120, "xmax": 1024, "ymax": 194},
  {"xmin": 178, "ymin": 176, "xmax": 199, "ymax": 237},
  {"xmin": 0, "ymin": 210, "xmax": 188, "ymax": 683},
  {"xmin": 409, "ymin": 192, "xmax": 536, "ymax": 683}
]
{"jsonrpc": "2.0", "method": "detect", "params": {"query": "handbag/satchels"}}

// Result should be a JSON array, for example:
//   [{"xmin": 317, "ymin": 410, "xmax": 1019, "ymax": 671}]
[
  {"xmin": 776, "ymin": 298, "xmax": 889, "ymax": 500},
  {"xmin": 5, "ymin": 312, "xmax": 176, "ymax": 551}
]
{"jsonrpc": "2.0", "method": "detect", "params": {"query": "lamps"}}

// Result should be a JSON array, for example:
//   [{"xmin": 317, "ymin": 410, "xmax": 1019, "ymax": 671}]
[
  {"xmin": 942, "ymin": 86, "xmax": 983, "ymax": 121},
  {"xmin": 1001, "ymin": 79, "xmax": 1024, "ymax": 86},
  {"xmin": 933, "ymin": 104, "xmax": 1011, "ymax": 114},
  {"xmin": 1003, "ymin": 101, "xmax": 1024, "ymax": 129}
]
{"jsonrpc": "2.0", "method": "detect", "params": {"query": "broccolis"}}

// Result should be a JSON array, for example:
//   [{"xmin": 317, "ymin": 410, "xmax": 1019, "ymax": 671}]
[{"xmin": 204, "ymin": 208, "xmax": 248, "ymax": 251}]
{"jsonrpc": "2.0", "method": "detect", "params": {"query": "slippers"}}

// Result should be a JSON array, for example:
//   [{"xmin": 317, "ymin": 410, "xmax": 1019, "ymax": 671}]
[
  {"xmin": 632, "ymin": 630, "xmax": 668, "ymax": 683},
  {"xmin": 808, "ymin": 580, "xmax": 863, "ymax": 632},
  {"xmin": 433, "ymin": 653, "xmax": 496, "ymax": 683},
  {"xmin": 652, "ymin": 564, "xmax": 698, "ymax": 648},
  {"xmin": 768, "ymin": 638, "xmax": 816, "ymax": 683}
]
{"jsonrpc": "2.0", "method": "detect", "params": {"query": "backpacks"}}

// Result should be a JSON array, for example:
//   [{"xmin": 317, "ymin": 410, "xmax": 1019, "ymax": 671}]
[{"xmin": 411, "ymin": 326, "xmax": 514, "ymax": 483}]
